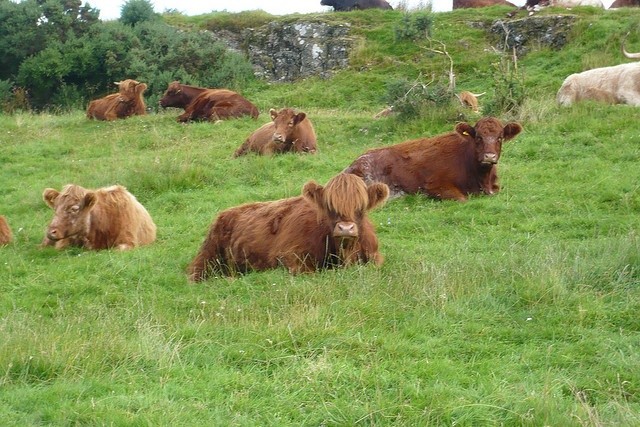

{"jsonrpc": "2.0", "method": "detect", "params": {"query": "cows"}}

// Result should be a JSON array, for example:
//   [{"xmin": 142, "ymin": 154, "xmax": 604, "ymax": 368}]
[
  {"xmin": 608, "ymin": 0, "xmax": 640, "ymax": 9},
  {"xmin": 452, "ymin": 0, "xmax": 518, "ymax": 10},
  {"xmin": 160, "ymin": 79, "xmax": 259, "ymax": 124},
  {"xmin": 41, "ymin": 183, "xmax": 157, "ymax": 254},
  {"xmin": 526, "ymin": 0, "xmax": 585, "ymax": 9},
  {"xmin": 321, "ymin": 0, "xmax": 394, "ymax": 12},
  {"xmin": 340, "ymin": 116, "xmax": 522, "ymax": 202},
  {"xmin": 450, "ymin": 91, "xmax": 487, "ymax": 117},
  {"xmin": 554, "ymin": 30, "xmax": 640, "ymax": 108},
  {"xmin": 86, "ymin": 78, "xmax": 148, "ymax": 121},
  {"xmin": 187, "ymin": 172, "xmax": 390, "ymax": 283},
  {"xmin": 232, "ymin": 107, "xmax": 318, "ymax": 158}
]
